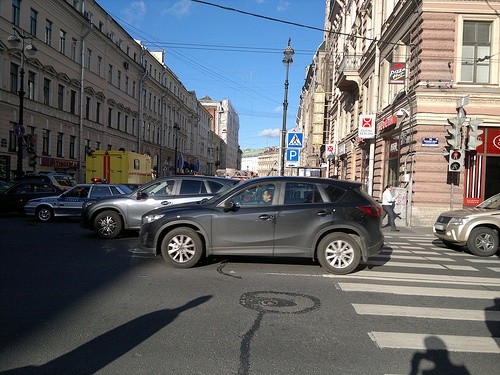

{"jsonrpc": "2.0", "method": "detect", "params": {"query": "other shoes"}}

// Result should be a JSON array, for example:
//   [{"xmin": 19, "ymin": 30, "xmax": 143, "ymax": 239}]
[{"xmin": 392, "ymin": 228, "xmax": 400, "ymax": 232}]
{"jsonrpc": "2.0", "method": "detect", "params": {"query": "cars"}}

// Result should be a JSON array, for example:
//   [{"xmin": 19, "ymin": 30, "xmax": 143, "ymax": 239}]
[
  {"xmin": 48, "ymin": 173, "xmax": 78, "ymax": 190},
  {"xmin": 0, "ymin": 180, "xmax": 78, "ymax": 213},
  {"xmin": 12, "ymin": 174, "xmax": 60, "ymax": 187},
  {"xmin": 433, "ymin": 192, "xmax": 500, "ymax": 257},
  {"xmin": 24, "ymin": 184, "xmax": 133, "ymax": 223}
]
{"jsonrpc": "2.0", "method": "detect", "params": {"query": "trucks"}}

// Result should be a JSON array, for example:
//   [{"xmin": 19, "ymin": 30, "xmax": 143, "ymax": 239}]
[{"xmin": 84, "ymin": 146, "xmax": 157, "ymax": 189}]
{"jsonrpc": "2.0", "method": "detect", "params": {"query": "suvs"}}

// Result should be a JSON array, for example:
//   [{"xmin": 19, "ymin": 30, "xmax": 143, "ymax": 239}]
[
  {"xmin": 139, "ymin": 177, "xmax": 384, "ymax": 275},
  {"xmin": 81, "ymin": 175, "xmax": 241, "ymax": 240}
]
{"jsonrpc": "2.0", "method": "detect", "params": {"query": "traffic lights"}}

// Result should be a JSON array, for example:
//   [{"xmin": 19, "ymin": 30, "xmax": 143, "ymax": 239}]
[
  {"xmin": 467, "ymin": 116, "xmax": 484, "ymax": 150},
  {"xmin": 449, "ymin": 149, "xmax": 465, "ymax": 171},
  {"xmin": 26, "ymin": 134, "xmax": 38, "ymax": 168},
  {"xmin": 446, "ymin": 116, "xmax": 465, "ymax": 150}
]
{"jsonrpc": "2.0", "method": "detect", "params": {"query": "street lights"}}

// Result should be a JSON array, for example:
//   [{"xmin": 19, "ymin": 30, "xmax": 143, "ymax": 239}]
[
  {"xmin": 279, "ymin": 36, "xmax": 294, "ymax": 176},
  {"xmin": 173, "ymin": 121, "xmax": 180, "ymax": 175},
  {"xmin": 7, "ymin": 27, "xmax": 38, "ymax": 181}
]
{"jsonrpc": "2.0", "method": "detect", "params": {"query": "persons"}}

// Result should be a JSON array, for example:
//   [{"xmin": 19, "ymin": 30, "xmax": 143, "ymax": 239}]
[
  {"xmin": 262, "ymin": 189, "xmax": 274, "ymax": 205},
  {"xmin": 382, "ymin": 184, "xmax": 401, "ymax": 231},
  {"xmin": 166, "ymin": 185, "xmax": 173, "ymax": 196}
]
